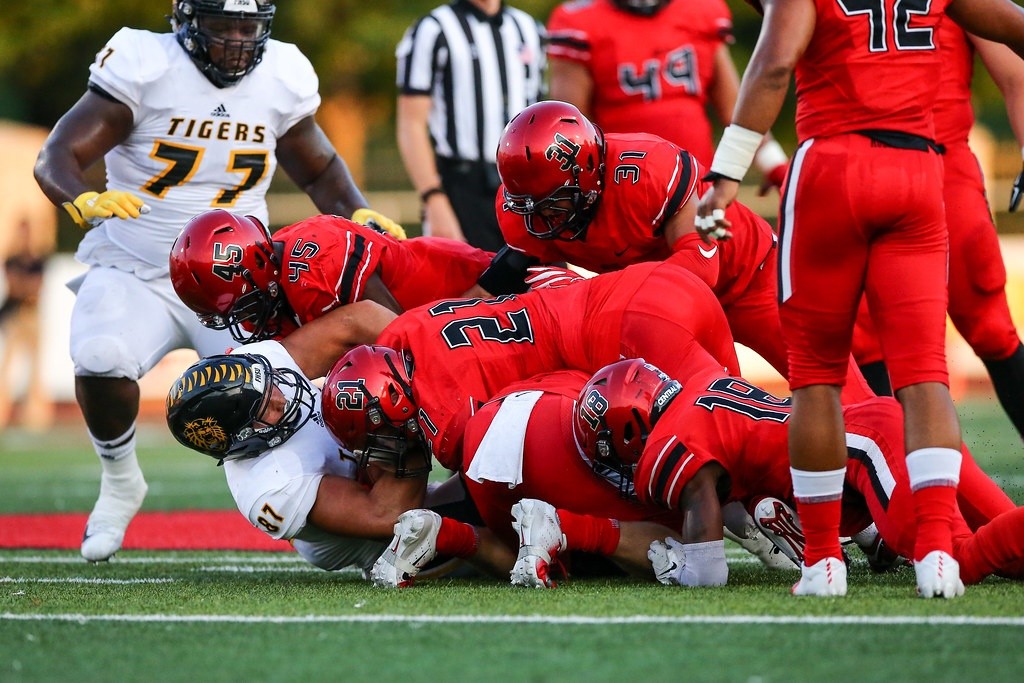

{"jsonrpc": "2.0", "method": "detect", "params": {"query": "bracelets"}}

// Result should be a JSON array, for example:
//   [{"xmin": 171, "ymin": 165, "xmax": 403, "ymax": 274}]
[{"xmin": 420, "ymin": 188, "xmax": 448, "ymax": 201}]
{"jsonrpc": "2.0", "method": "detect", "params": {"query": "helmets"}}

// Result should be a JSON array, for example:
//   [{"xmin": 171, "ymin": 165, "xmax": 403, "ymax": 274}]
[
  {"xmin": 168, "ymin": 208, "xmax": 285, "ymax": 346},
  {"xmin": 164, "ymin": 0, "xmax": 277, "ymax": 88},
  {"xmin": 164, "ymin": 353, "xmax": 315, "ymax": 468},
  {"xmin": 321, "ymin": 344, "xmax": 432, "ymax": 476},
  {"xmin": 497, "ymin": 100, "xmax": 607, "ymax": 242},
  {"xmin": 572, "ymin": 356, "xmax": 683, "ymax": 501}
]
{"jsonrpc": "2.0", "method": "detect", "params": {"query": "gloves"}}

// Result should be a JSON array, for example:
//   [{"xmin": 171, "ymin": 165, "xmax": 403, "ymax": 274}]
[
  {"xmin": 61, "ymin": 189, "xmax": 153, "ymax": 228},
  {"xmin": 351, "ymin": 207, "xmax": 408, "ymax": 242},
  {"xmin": 648, "ymin": 536, "xmax": 688, "ymax": 587}
]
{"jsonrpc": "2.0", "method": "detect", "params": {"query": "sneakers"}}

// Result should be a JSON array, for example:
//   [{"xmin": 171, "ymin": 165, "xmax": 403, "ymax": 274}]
[
  {"xmin": 914, "ymin": 550, "xmax": 964, "ymax": 599},
  {"xmin": 80, "ymin": 470, "xmax": 147, "ymax": 566},
  {"xmin": 789, "ymin": 557, "xmax": 847, "ymax": 597},
  {"xmin": 369, "ymin": 508, "xmax": 442, "ymax": 590},
  {"xmin": 857, "ymin": 533, "xmax": 900, "ymax": 573},
  {"xmin": 724, "ymin": 513, "xmax": 800, "ymax": 571},
  {"xmin": 510, "ymin": 498, "xmax": 568, "ymax": 589},
  {"xmin": 750, "ymin": 493, "xmax": 852, "ymax": 573}
]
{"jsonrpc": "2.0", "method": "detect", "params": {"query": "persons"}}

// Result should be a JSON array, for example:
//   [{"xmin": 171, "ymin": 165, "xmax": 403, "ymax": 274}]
[
  {"xmin": 544, "ymin": 0, "xmax": 791, "ymax": 195},
  {"xmin": 168, "ymin": 101, "xmax": 1024, "ymax": 591},
  {"xmin": 394, "ymin": 0, "xmax": 549, "ymax": 253},
  {"xmin": 33, "ymin": 0, "xmax": 405, "ymax": 564},
  {"xmin": 696, "ymin": 0, "xmax": 1024, "ymax": 600}
]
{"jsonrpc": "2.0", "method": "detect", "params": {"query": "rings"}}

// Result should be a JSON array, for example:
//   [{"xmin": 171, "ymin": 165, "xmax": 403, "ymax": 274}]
[
  {"xmin": 700, "ymin": 219, "xmax": 709, "ymax": 230},
  {"xmin": 707, "ymin": 231, "xmax": 719, "ymax": 239},
  {"xmin": 705, "ymin": 215, "xmax": 716, "ymax": 227},
  {"xmin": 714, "ymin": 227, "xmax": 726, "ymax": 238},
  {"xmin": 694, "ymin": 215, "xmax": 702, "ymax": 227},
  {"xmin": 712, "ymin": 209, "xmax": 724, "ymax": 221}
]
